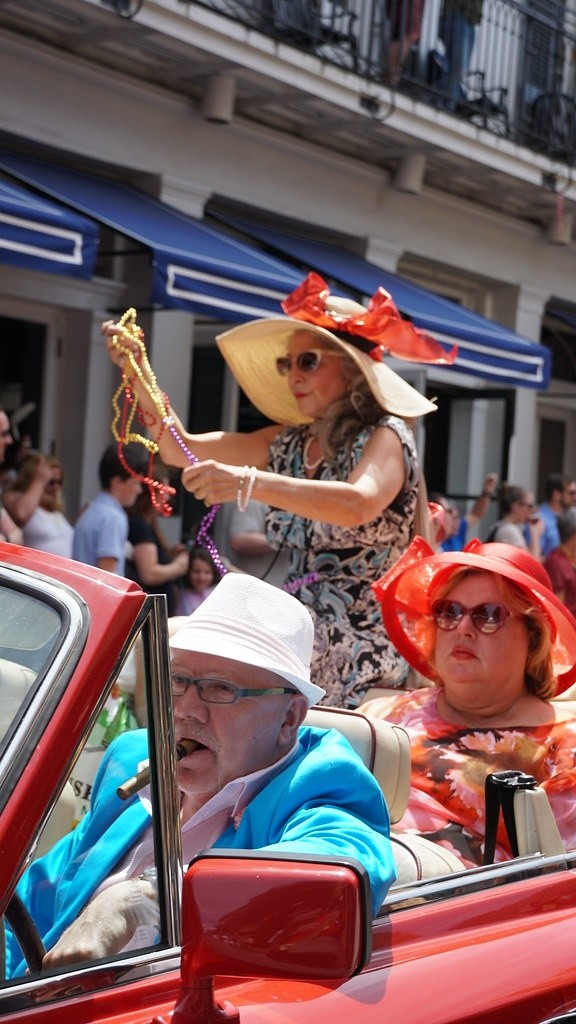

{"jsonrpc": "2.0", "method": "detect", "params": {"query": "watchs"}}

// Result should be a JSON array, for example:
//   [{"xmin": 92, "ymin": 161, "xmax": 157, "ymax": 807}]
[{"xmin": 139, "ymin": 867, "xmax": 156, "ymax": 888}]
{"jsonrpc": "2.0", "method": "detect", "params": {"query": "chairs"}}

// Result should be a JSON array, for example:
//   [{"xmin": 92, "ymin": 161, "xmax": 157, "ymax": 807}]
[
  {"xmin": 301, "ymin": 703, "xmax": 472, "ymax": 889},
  {"xmin": 430, "ymin": 50, "xmax": 512, "ymax": 138},
  {"xmin": 267, "ymin": 0, "xmax": 357, "ymax": 72},
  {"xmin": 532, "ymin": 93, "xmax": 576, "ymax": 168}
]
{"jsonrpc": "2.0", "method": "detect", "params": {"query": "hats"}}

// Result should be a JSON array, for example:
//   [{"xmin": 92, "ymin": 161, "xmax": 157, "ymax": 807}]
[
  {"xmin": 166, "ymin": 573, "xmax": 327, "ymax": 707},
  {"xmin": 370, "ymin": 535, "xmax": 576, "ymax": 698},
  {"xmin": 215, "ymin": 271, "xmax": 458, "ymax": 429}
]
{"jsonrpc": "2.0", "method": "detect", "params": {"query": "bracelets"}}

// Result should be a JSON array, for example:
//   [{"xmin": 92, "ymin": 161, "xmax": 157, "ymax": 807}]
[
  {"xmin": 482, "ymin": 491, "xmax": 489, "ymax": 496},
  {"xmin": 237, "ymin": 465, "xmax": 256, "ymax": 512}
]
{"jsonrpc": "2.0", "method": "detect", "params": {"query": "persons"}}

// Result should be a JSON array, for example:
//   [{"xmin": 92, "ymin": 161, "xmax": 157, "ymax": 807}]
[
  {"xmin": 385, "ymin": 0, "xmax": 485, "ymax": 112},
  {"xmin": 223, "ymin": 501, "xmax": 290, "ymax": 586},
  {"xmin": 3, "ymin": 573, "xmax": 395, "ymax": 978},
  {"xmin": 102, "ymin": 271, "xmax": 459, "ymax": 709},
  {"xmin": 427, "ymin": 472, "xmax": 576, "ymax": 621},
  {"xmin": 1, "ymin": 409, "xmax": 216, "ymax": 616},
  {"xmin": 353, "ymin": 536, "xmax": 576, "ymax": 863}
]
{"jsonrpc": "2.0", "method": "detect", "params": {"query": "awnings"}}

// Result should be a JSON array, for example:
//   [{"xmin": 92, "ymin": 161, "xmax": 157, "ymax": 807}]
[
  {"xmin": 0, "ymin": 176, "xmax": 98, "ymax": 282},
  {"xmin": 0, "ymin": 150, "xmax": 354, "ymax": 323},
  {"xmin": 203, "ymin": 205, "xmax": 550, "ymax": 389}
]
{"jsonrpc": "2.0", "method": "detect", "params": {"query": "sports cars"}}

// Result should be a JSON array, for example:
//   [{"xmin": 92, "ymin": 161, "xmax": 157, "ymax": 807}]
[{"xmin": 0, "ymin": 542, "xmax": 576, "ymax": 1024}]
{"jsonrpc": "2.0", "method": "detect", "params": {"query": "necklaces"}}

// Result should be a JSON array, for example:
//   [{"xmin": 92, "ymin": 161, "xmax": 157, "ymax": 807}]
[
  {"xmin": 303, "ymin": 437, "xmax": 324, "ymax": 469},
  {"xmin": 111, "ymin": 308, "xmax": 229, "ymax": 578}
]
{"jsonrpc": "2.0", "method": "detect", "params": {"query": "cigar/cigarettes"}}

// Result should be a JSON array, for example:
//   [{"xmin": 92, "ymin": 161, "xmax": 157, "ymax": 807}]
[{"xmin": 118, "ymin": 740, "xmax": 198, "ymax": 801}]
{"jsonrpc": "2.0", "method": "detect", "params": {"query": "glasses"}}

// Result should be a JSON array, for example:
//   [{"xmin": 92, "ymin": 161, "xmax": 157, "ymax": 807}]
[
  {"xmin": 432, "ymin": 596, "xmax": 528, "ymax": 634},
  {"xmin": 515, "ymin": 503, "xmax": 532, "ymax": 509},
  {"xmin": 45, "ymin": 479, "xmax": 63, "ymax": 488},
  {"xmin": 275, "ymin": 349, "xmax": 351, "ymax": 377},
  {"xmin": 169, "ymin": 670, "xmax": 300, "ymax": 705},
  {"xmin": 0, "ymin": 429, "xmax": 10, "ymax": 437},
  {"xmin": 559, "ymin": 490, "xmax": 576, "ymax": 496},
  {"xmin": 442, "ymin": 507, "xmax": 453, "ymax": 515}
]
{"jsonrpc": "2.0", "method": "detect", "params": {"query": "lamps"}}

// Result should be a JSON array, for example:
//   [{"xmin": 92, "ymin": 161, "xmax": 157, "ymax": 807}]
[
  {"xmin": 545, "ymin": 210, "xmax": 574, "ymax": 244},
  {"xmin": 201, "ymin": 69, "xmax": 235, "ymax": 122},
  {"xmin": 392, "ymin": 146, "xmax": 427, "ymax": 193}
]
